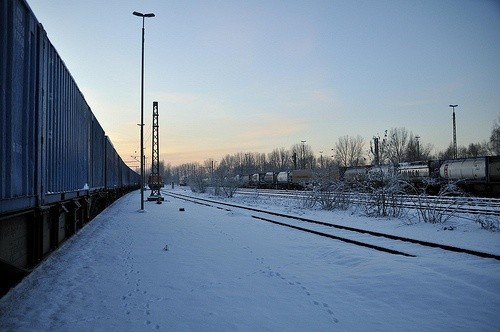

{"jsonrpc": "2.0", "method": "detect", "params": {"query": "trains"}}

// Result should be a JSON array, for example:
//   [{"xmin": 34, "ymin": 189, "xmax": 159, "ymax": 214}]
[
  {"xmin": 338, "ymin": 153, "xmax": 500, "ymax": 198},
  {"xmin": 203, "ymin": 168, "xmax": 314, "ymax": 190}
]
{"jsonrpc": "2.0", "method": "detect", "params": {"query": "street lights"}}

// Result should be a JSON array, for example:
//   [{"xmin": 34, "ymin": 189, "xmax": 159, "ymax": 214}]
[
  {"xmin": 301, "ymin": 140, "xmax": 307, "ymax": 169},
  {"xmin": 415, "ymin": 136, "xmax": 421, "ymax": 159},
  {"xmin": 449, "ymin": 104, "xmax": 459, "ymax": 160},
  {"xmin": 131, "ymin": 10, "xmax": 156, "ymax": 215}
]
